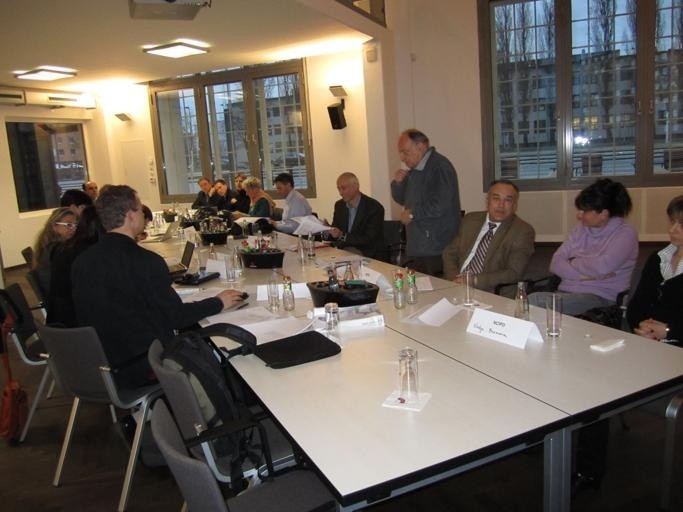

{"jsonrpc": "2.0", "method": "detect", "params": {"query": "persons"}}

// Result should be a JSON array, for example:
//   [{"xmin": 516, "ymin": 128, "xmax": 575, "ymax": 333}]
[
  {"xmin": 391, "ymin": 129, "xmax": 461, "ymax": 278},
  {"xmin": 441, "ymin": 179, "xmax": 535, "ymax": 300},
  {"xmin": 31, "ymin": 206, "xmax": 80, "ymax": 295},
  {"xmin": 270, "ymin": 173, "xmax": 312, "ymax": 234},
  {"xmin": 70, "ymin": 185, "xmax": 244, "ymax": 463},
  {"xmin": 223, "ymin": 174, "xmax": 250, "ymax": 214},
  {"xmin": 626, "ymin": 194, "xmax": 683, "ymax": 348},
  {"xmin": 318, "ymin": 172, "xmax": 384, "ymax": 258},
  {"xmin": 45, "ymin": 206, "xmax": 106, "ymax": 327},
  {"xmin": 82, "ymin": 181, "xmax": 98, "ymax": 200},
  {"xmin": 61, "ymin": 189, "xmax": 92, "ymax": 214},
  {"xmin": 230, "ymin": 176, "xmax": 274, "ymax": 220},
  {"xmin": 135, "ymin": 205, "xmax": 153, "ymax": 241},
  {"xmin": 191, "ymin": 176, "xmax": 218, "ymax": 209},
  {"xmin": 206, "ymin": 179, "xmax": 239, "ymax": 212},
  {"xmin": 527, "ymin": 178, "xmax": 639, "ymax": 317}
]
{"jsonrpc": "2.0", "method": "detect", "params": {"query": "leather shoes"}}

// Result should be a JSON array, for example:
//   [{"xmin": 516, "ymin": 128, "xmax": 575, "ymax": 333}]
[{"xmin": 120, "ymin": 414, "xmax": 137, "ymax": 444}]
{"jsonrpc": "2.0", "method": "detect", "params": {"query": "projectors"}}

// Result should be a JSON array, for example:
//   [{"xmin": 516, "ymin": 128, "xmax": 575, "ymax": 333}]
[{"xmin": 128, "ymin": 0, "xmax": 205, "ymax": 20}]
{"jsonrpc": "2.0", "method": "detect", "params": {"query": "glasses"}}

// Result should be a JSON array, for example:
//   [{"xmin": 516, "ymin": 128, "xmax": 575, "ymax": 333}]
[{"xmin": 56, "ymin": 222, "xmax": 77, "ymax": 228}]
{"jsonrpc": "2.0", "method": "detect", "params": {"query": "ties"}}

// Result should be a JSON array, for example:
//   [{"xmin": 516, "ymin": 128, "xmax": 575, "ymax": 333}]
[{"xmin": 463, "ymin": 222, "xmax": 496, "ymax": 273}]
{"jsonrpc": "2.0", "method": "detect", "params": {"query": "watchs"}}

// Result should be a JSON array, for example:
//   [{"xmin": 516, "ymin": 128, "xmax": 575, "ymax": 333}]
[{"xmin": 409, "ymin": 213, "xmax": 414, "ymax": 220}]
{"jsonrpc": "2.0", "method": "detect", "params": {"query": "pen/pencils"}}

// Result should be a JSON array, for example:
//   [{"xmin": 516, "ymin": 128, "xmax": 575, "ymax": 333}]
[{"xmin": 234, "ymin": 302, "xmax": 249, "ymax": 310}]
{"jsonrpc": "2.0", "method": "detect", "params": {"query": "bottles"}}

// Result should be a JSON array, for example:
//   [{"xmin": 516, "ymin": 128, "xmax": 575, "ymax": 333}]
[
  {"xmin": 154, "ymin": 212, "xmax": 158, "ymax": 230},
  {"xmin": 297, "ymin": 235, "xmax": 306, "ymax": 265},
  {"xmin": 307, "ymin": 231, "xmax": 315, "ymax": 256},
  {"xmin": 200, "ymin": 219, "xmax": 227, "ymax": 231},
  {"xmin": 242, "ymin": 220, "xmax": 248, "ymax": 238},
  {"xmin": 282, "ymin": 276, "xmax": 294, "ymax": 310},
  {"xmin": 515, "ymin": 282, "xmax": 529, "ymax": 320},
  {"xmin": 209, "ymin": 243, "xmax": 216, "ymax": 260},
  {"xmin": 329, "ymin": 270, "xmax": 339, "ymax": 291},
  {"xmin": 343, "ymin": 264, "xmax": 354, "ymax": 280},
  {"xmin": 330, "ymin": 257, "xmax": 336, "ymax": 275},
  {"xmin": 258, "ymin": 231, "xmax": 262, "ymax": 247}
]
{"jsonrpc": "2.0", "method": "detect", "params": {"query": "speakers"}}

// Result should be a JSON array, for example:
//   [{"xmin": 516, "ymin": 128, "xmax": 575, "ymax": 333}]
[{"xmin": 327, "ymin": 103, "xmax": 346, "ymax": 130}]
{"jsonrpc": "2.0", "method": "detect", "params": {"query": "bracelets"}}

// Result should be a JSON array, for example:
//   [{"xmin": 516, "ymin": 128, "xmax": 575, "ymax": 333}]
[{"xmin": 666, "ymin": 323, "xmax": 672, "ymax": 340}]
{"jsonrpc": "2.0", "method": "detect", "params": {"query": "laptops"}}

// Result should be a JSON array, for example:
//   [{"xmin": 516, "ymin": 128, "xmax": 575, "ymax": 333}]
[
  {"xmin": 142, "ymin": 221, "xmax": 179, "ymax": 242},
  {"xmin": 169, "ymin": 241, "xmax": 195, "ymax": 276}
]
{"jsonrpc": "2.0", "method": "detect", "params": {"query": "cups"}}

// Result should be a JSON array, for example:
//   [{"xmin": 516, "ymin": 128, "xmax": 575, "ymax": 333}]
[
  {"xmin": 177, "ymin": 228, "xmax": 181, "ymax": 240},
  {"xmin": 393, "ymin": 269, "xmax": 406, "ymax": 308},
  {"xmin": 267, "ymin": 277, "xmax": 280, "ymax": 312},
  {"xmin": 545, "ymin": 294, "xmax": 562, "ymax": 336},
  {"xmin": 405, "ymin": 268, "xmax": 416, "ymax": 304},
  {"xmin": 463, "ymin": 272, "xmax": 474, "ymax": 305},
  {"xmin": 399, "ymin": 348, "xmax": 418, "ymax": 403},
  {"xmin": 227, "ymin": 236, "xmax": 233, "ymax": 247},
  {"xmin": 198, "ymin": 247, "xmax": 207, "ymax": 269},
  {"xmin": 224, "ymin": 255, "xmax": 235, "ymax": 281},
  {"xmin": 325, "ymin": 303, "xmax": 342, "ymax": 347}
]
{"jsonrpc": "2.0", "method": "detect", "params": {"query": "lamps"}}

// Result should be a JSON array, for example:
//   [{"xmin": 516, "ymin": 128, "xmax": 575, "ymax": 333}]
[
  {"xmin": 144, "ymin": 43, "xmax": 208, "ymax": 58},
  {"xmin": 15, "ymin": 69, "xmax": 76, "ymax": 81}
]
